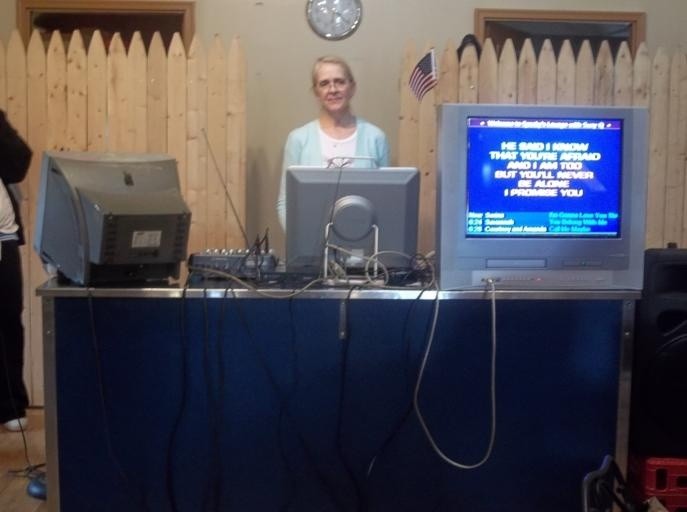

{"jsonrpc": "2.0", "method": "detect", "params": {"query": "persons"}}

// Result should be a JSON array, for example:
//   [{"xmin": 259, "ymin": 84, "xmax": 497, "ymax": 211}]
[
  {"xmin": 0, "ymin": 108, "xmax": 33, "ymax": 431},
  {"xmin": 277, "ymin": 54, "xmax": 389, "ymax": 237}
]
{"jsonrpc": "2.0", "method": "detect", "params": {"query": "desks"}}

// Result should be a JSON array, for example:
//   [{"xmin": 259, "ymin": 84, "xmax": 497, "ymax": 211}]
[{"xmin": 35, "ymin": 258, "xmax": 641, "ymax": 512}]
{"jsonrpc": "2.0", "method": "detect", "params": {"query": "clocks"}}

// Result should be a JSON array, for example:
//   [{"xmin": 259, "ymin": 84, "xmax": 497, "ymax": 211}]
[{"xmin": 306, "ymin": 0, "xmax": 362, "ymax": 40}]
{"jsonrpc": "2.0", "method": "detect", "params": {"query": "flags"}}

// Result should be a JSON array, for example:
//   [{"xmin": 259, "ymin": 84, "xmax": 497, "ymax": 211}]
[{"xmin": 405, "ymin": 52, "xmax": 438, "ymax": 102}]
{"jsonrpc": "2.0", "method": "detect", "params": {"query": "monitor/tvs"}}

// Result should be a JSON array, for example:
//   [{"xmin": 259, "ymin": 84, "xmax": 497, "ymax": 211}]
[
  {"xmin": 284, "ymin": 164, "xmax": 422, "ymax": 271},
  {"xmin": 435, "ymin": 100, "xmax": 650, "ymax": 291},
  {"xmin": 32, "ymin": 148, "xmax": 192, "ymax": 287}
]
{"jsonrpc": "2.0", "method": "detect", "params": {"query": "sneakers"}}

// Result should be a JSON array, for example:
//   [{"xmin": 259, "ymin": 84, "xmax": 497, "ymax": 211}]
[{"xmin": 5, "ymin": 418, "xmax": 27, "ymax": 431}]
